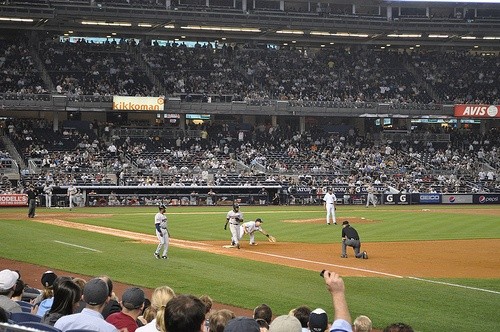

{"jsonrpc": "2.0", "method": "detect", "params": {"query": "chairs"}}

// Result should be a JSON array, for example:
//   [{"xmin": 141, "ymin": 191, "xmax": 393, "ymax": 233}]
[{"xmin": 0, "ymin": 300, "xmax": 95, "ymax": 332}]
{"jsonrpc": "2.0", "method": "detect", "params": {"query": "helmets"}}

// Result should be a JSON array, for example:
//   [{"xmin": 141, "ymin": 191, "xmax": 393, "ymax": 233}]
[
  {"xmin": 233, "ymin": 203, "xmax": 240, "ymax": 212},
  {"xmin": 158, "ymin": 204, "xmax": 167, "ymax": 210}
]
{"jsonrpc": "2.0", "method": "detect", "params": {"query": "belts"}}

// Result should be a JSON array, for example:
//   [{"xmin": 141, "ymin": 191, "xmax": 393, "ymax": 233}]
[
  {"xmin": 230, "ymin": 223, "xmax": 238, "ymax": 225},
  {"xmin": 330, "ymin": 203, "xmax": 333, "ymax": 204}
]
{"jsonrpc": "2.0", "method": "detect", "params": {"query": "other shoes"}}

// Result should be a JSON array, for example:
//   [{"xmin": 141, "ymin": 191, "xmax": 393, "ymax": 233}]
[
  {"xmin": 327, "ymin": 223, "xmax": 330, "ymax": 225},
  {"xmin": 231, "ymin": 242, "xmax": 234, "ymax": 246},
  {"xmin": 236, "ymin": 243, "xmax": 241, "ymax": 249},
  {"xmin": 341, "ymin": 254, "xmax": 347, "ymax": 258},
  {"xmin": 334, "ymin": 223, "xmax": 337, "ymax": 225},
  {"xmin": 154, "ymin": 253, "xmax": 161, "ymax": 260},
  {"xmin": 162, "ymin": 255, "xmax": 167, "ymax": 259},
  {"xmin": 363, "ymin": 251, "xmax": 368, "ymax": 259},
  {"xmin": 249, "ymin": 243, "xmax": 258, "ymax": 246}
]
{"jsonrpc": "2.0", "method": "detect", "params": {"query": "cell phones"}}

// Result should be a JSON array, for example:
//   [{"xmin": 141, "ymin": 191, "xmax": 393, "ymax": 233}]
[{"xmin": 320, "ymin": 270, "xmax": 330, "ymax": 277}]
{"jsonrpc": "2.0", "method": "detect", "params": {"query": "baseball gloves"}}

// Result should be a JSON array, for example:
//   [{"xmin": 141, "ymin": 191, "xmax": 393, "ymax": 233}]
[{"xmin": 266, "ymin": 236, "xmax": 277, "ymax": 243}]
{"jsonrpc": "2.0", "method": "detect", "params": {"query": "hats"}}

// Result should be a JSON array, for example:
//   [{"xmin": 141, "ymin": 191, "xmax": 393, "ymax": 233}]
[
  {"xmin": 309, "ymin": 308, "xmax": 328, "ymax": 332},
  {"xmin": 223, "ymin": 317, "xmax": 261, "ymax": 332},
  {"xmin": 328, "ymin": 188, "xmax": 332, "ymax": 191},
  {"xmin": 83, "ymin": 276, "xmax": 113, "ymax": 306},
  {"xmin": 122, "ymin": 288, "xmax": 145, "ymax": 310},
  {"xmin": 40, "ymin": 271, "xmax": 58, "ymax": 288},
  {"xmin": 0, "ymin": 269, "xmax": 19, "ymax": 290},
  {"xmin": 255, "ymin": 218, "xmax": 264, "ymax": 223},
  {"xmin": 342, "ymin": 221, "xmax": 349, "ymax": 225}
]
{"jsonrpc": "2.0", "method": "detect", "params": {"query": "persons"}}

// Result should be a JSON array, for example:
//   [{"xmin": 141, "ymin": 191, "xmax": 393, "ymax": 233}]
[
  {"xmin": 43, "ymin": 183, "xmax": 53, "ymax": 208},
  {"xmin": 224, "ymin": 204, "xmax": 243, "ymax": 249},
  {"xmin": 0, "ymin": 119, "xmax": 500, "ymax": 204},
  {"xmin": 153, "ymin": 205, "xmax": 171, "ymax": 259},
  {"xmin": 366, "ymin": 185, "xmax": 377, "ymax": 208},
  {"xmin": 322, "ymin": 187, "xmax": 337, "ymax": 225},
  {"xmin": 231, "ymin": 218, "xmax": 276, "ymax": 245},
  {"xmin": 26, "ymin": 186, "xmax": 39, "ymax": 218},
  {"xmin": 67, "ymin": 183, "xmax": 80, "ymax": 208},
  {"xmin": 340, "ymin": 221, "xmax": 368, "ymax": 259},
  {"xmin": 0, "ymin": 269, "xmax": 415, "ymax": 332},
  {"xmin": 0, "ymin": 31, "xmax": 500, "ymax": 109}
]
